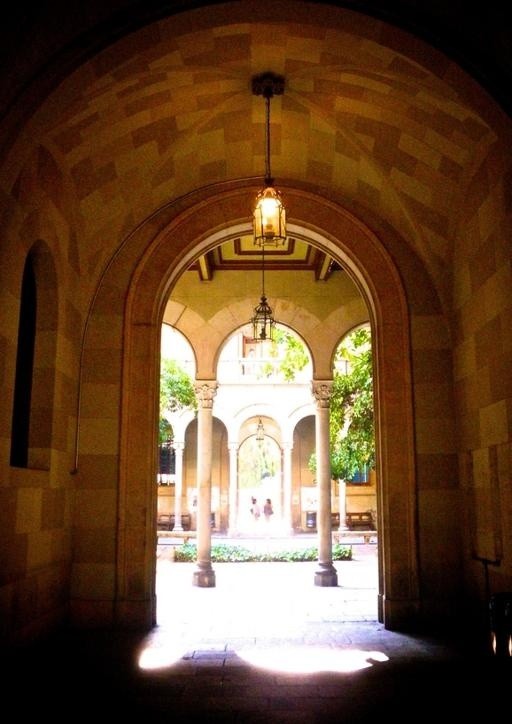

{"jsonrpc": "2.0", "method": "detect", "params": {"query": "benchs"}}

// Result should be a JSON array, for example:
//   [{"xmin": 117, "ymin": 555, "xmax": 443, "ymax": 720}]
[
  {"xmin": 330, "ymin": 512, "xmax": 376, "ymax": 531},
  {"xmin": 157, "ymin": 514, "xmax": 192, "ymax": 529}
]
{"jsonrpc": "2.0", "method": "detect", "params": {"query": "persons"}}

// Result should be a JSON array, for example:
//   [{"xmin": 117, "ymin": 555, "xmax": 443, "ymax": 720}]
[
  {"xmin": 263, "ymin": 497, "xmax": 273, "ymax": 519},
  {"xmin": 249, "ymin": 497, "xmax": 261, "ymax": 520}
]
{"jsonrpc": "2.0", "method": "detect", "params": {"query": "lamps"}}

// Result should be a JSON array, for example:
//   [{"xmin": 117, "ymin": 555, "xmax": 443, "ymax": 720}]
[
  {"xmin": 255, "ymin": 416, "xmax": 265, "ymax": 446},
  {"xmin": 250, "ymin": 69, "xmax": 288, "ymax": 249},
  {"xmin": 251, "ymin": 246, "xmax": 275, "ymax": 343}
]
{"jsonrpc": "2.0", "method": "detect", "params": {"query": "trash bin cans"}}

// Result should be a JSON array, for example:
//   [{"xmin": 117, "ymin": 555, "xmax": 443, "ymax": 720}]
[{"xmin": 306, "ymin": 511, "xmax": 317, "ymax": 528}]
